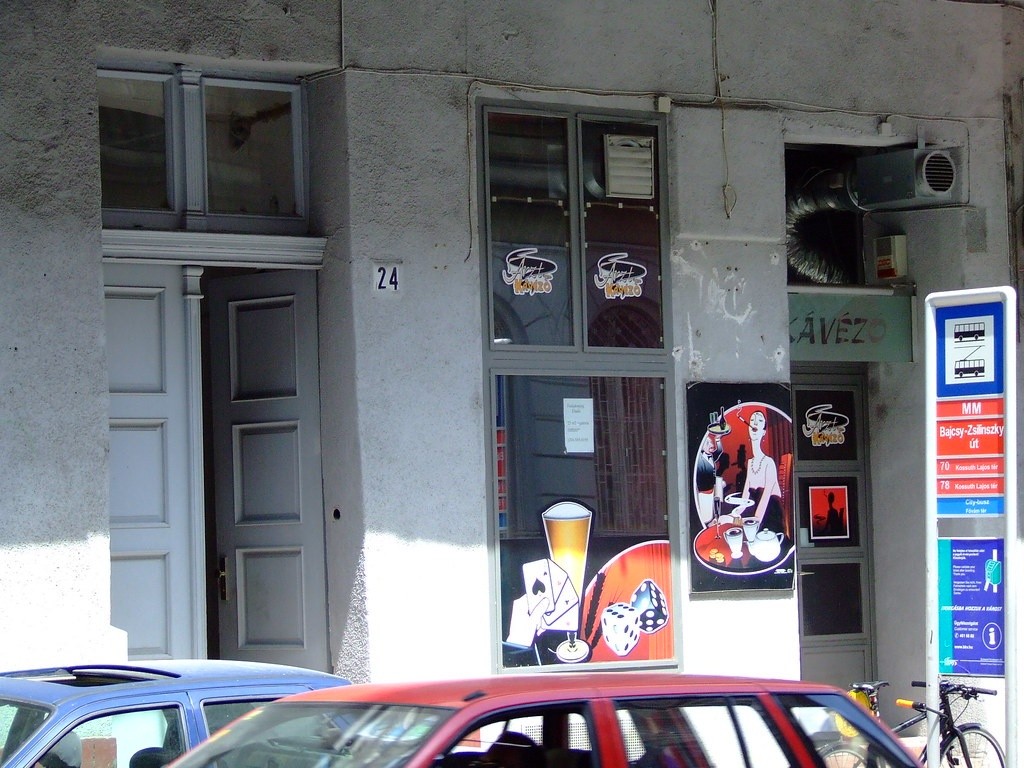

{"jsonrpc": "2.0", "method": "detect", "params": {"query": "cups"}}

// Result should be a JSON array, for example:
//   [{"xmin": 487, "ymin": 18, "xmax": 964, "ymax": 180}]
[
  {"xmin": 723, "ymin": 527, "xmax": 743, "ymax": 559},
  {"xmin": 742, "ymin": 519, "xmax": 759, "ymax": 542}
]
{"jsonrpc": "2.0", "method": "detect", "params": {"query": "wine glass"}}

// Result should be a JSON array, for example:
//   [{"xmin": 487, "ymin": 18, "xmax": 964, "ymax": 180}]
[
  {"xmin": 542, "ymin": 513, "xmax": 592, "ymax": 663},
  {"xmin": 713, "ymin": 497, "xmax": 721, "ymax": 539}
]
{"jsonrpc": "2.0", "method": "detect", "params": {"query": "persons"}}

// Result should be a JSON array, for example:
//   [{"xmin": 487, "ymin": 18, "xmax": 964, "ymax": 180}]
[{"xmin": 482, "ymin": 732, "xmax": 547, "ymax": 768}]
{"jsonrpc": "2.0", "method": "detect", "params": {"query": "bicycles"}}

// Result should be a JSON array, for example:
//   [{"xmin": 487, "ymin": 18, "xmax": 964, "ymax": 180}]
[{"xmin": 815, "ymin": 679, "xmax": 1008, "ymax": 768}]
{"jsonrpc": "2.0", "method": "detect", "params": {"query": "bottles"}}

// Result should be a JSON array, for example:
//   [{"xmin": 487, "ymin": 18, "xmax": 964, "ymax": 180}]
[{"xmin": 720, "ymin": 407, "xmax": 726, "ymax": 430}]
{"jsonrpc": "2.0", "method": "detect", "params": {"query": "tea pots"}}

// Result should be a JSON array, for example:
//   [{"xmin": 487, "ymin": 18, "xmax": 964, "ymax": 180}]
[{"xmin": 743, "ymin": 528, "xmax": 784, "ymax": 562}]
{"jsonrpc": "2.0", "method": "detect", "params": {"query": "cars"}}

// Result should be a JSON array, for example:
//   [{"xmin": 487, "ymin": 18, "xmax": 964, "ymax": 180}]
[
  {"xmin": 2, "ymin": 659, "xmax": 418, "ymax": 768},
  {"xmin": 158, "ymin": 669, "xmax": 924, "ymax": 768}
]
